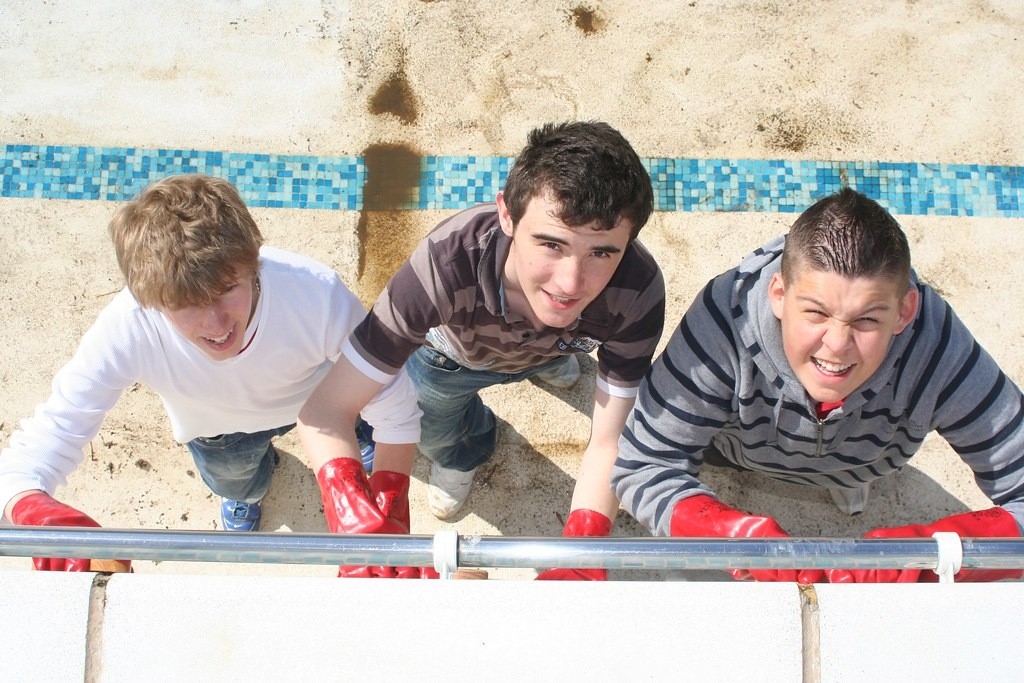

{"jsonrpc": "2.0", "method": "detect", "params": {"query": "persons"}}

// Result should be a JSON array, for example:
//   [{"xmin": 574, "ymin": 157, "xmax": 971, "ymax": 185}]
[
  {"xmin": 299, "ymin": 121, "xmax": 665, "ymax": 579},
  {"xmin": 0, "ymin": 175, "xmax": 425, "ymax": 573},
  {"xmin": 610, "ymin": 189, "xmax": 1024, "ymax": 583}
]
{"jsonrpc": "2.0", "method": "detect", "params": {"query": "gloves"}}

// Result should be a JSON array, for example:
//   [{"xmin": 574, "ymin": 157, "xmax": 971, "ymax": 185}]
[
  {"xmin": 12, "ymin": 493, "xmax": 131, "ymax": 572},
  {"xmin": 825, "ymin": 504, "xmax": 1024, "ymax": 583},
  {"xmin": 318, "ymin": 456, "xmax": 441, "ymax": 579},
  {"xmin": 534, "ymin": 508, "xmax": 612, "ymax": 581},
  {"xmin": 670, "ymin": 494, "xmax": 824, "ymax": 584}
]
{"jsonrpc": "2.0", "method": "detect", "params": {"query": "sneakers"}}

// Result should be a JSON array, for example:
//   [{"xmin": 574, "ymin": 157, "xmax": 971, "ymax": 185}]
[
  {"xmin": 222, "ymin": 496, "xmax": 261, "ymax": 533},
  {"xmin": 429, "ymin": 461, "xmax": 476, "ymax": 517},
  {"xmin": 355, "ymin": 420, "xmax": 376, "ymax": 471},
  {"xmin": 831, "ymin": 483, "xmax": 869, "ymax": 515},
  {"xmin": 537, "ymin": 354, "xmax": 581, "ymax": 389}
]
